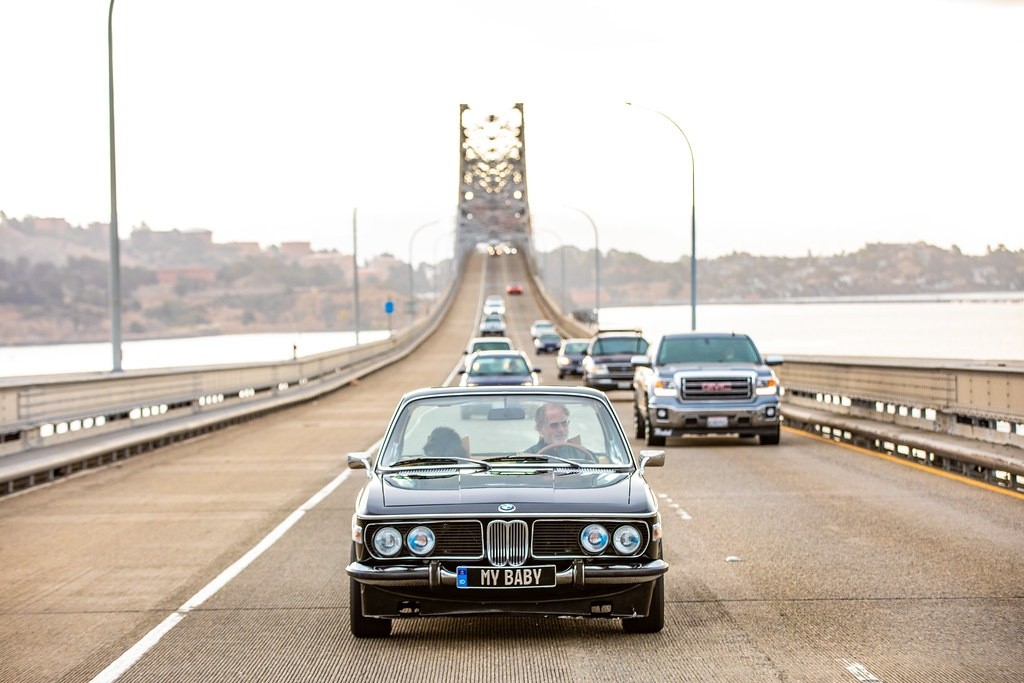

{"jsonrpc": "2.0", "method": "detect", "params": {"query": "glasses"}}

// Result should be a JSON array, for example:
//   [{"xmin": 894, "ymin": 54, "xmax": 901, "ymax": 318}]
[{"xmin": 544, "ymin": 418, "xmax": 570, "ymax": 429}]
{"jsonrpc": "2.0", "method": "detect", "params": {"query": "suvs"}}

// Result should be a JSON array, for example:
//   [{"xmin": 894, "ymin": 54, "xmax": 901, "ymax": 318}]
[
  {"xmin": 479, "ymin": 315, "xmax": 507, "ymax": 338},
  {"xmin": 581, "ymin": 329, "xmax": 650, "ymax": 392},
  {"xmin": 631, "ymin": 330, "xmax": 786, "ymax": 447},
  {"xmin": 482, "ymin": 302, "xmax": 507, "ymax": 315}
]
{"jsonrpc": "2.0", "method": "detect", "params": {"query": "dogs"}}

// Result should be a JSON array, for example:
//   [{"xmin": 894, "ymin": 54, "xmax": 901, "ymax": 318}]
[{"xmin": 423, "ymin": 427, "xmax": 469, "ymax": 465}]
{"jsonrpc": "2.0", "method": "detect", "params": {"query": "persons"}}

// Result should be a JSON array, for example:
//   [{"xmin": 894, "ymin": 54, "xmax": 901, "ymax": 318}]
[
  {"xmin": 521, "ymin": 400, "xmax": 596, "ymax": 462},
  {"xmin": 507, "ymin": 361, "xmax": 519, "ymax": 372}
]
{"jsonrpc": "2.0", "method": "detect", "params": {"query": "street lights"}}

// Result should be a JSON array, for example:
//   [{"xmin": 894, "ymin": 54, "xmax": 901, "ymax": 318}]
[
  {"xmin": 619, "ymin": 99, "xmax": 698, "ymax": 328},
  {"xmin": 564, "ymin": 203, "xmax": 600, "ymax": 328}
]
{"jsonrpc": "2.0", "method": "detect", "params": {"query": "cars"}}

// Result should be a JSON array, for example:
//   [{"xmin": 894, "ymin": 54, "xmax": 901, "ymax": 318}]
[
  {"xmin": 556, "ymin": 338, "xmax": 591, "ymax": 380},
  {"xmin": 484, "ymin": 294, "xmax": 504, "ymax": 302},
  {"xmin": 505, "ymin": 281, "xmax": 524, "ymax": 295},
  {"xmin": 534, "ymin": 332, "xmax": 563, "ymax": 355},
  {"xmin": 458, "ymin": 350, "xmax": 543, "ymax": 390},
  {"xmin": 346, "ymin": 384, "xmax": 669, "ymax": 638},
  {"xmin": 529, "ymin": 320, "xmax": 555, "ymax": 340},
  {"xmin": 463, "ymin": 337, "xmax": 516, "ymax": 356}
]
